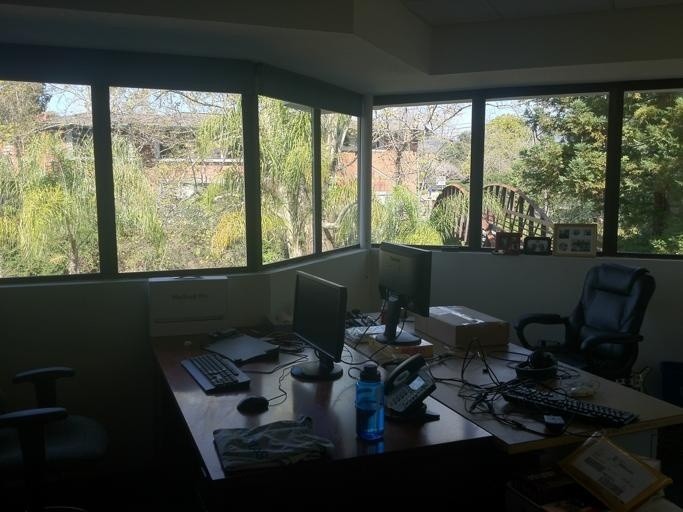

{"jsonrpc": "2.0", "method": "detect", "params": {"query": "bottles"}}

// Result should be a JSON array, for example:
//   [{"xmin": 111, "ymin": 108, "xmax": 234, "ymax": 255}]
[{"xmin": 354, "ymin": 363, "xmax": 386, "ymax": 442}]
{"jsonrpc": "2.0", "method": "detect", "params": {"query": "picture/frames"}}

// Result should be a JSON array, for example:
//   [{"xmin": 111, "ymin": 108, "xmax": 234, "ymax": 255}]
[
  {"xmin": 553, "ymin": 224, "xmax": 597, "ymax": 257},
  {"xmin": 524, "ymin": 237, "xmax": 551, "ymax": 255}
]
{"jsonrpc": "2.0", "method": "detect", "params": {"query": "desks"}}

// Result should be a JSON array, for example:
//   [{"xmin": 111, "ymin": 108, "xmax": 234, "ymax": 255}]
[
  {"xmin": 151, "ymin": 325, "xmax": 493, "ymax": 512},
  {"xmin": 344, "ymin": 311, "xmax": 682, "ymax": 454}
]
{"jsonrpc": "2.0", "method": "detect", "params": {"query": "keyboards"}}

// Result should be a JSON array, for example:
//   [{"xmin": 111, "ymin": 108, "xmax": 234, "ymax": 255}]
[
  {"xmin": 502, "ymin": 384, "xmax": 639, "ymax": 428},
  {"xmin": 180, "ymin": 352, "xmax": 251, "ymax": 393}
]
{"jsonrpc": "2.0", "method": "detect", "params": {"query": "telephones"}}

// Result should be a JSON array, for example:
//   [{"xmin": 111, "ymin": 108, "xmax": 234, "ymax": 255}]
[{"xmin": 376, "ymin": 352, "xmax": 437, "ymax": 415}]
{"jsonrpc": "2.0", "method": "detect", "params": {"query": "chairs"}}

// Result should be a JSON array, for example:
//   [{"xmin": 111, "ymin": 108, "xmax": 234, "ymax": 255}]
[
  {"xmin": 515, "ymin": 263, "xmax": 656, "ymax": 384},
  {"xmin": 1, "ymin": 365, "xmax": 116, "ymax": 512}
]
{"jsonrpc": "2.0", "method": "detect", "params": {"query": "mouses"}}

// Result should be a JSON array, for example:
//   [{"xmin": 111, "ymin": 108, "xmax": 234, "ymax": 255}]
[
  {"xmin": 237, "ymin": 395, "xmax": 269, "ymax": 413},
  {"xmin": 569, "ymin": 385, "xmax": 593, "ymax": 398}
]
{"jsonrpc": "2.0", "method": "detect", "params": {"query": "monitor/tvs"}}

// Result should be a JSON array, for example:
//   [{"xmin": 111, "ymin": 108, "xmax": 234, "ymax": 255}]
[
  {"xmin": 290, "ymin": 271, "xmax": 347, "ymax": 382},
  {"xmin": 376, "ymin": 242, "xmax": 433, "ymax": 345}
]
